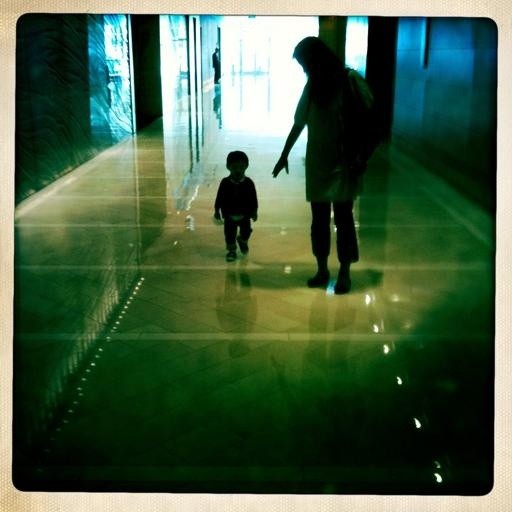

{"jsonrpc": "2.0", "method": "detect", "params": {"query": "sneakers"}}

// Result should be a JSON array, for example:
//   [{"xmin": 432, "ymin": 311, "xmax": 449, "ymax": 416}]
[
  {"xmin": 227, "ymin": 252, "xmax": 236, "ymax": 260},
  {"xmin": 238, "ymin": 236, "xmax": 248, "ymax": 254}
]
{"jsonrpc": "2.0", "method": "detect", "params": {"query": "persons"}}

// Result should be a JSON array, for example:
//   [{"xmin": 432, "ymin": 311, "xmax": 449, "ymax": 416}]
[
  {"xmin": 214, "ymin": 151, "xmax": 258, "ymax": 261},
  {"xmin": 212, "ymin": 48, "xmax": 221, "ymax": 84},
  {"xmin": 271, "ymin": 38, "xmax": 379, "ymax": 294}
]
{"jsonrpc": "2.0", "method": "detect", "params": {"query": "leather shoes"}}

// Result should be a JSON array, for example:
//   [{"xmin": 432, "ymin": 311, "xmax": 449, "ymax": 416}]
[
  {"xmin": 336, "ymin": 276, "xmax": 351, "ymax": 293},
  {"xmin": 308, "ymin": 271, "xmax": 329, "ymax": 286}
]
